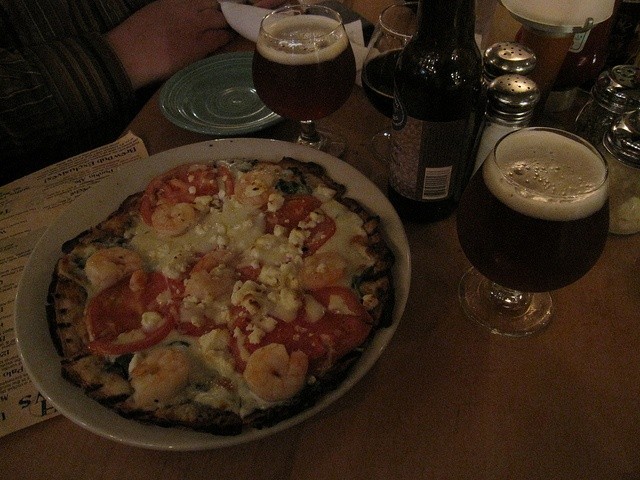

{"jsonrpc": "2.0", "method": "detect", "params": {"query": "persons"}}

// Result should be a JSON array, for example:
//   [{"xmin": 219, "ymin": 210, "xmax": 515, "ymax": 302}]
[{"xmin": 0, "ymin": 0, "xmax": 235, "ymax": 176}]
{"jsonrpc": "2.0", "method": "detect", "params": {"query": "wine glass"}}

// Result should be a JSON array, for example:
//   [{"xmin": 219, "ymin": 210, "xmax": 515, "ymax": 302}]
[
  {"xmin": 455, "ymin": 127, "xmax": 612, "ymax": 341},
  {"xmin": 251, "ymin": 4, "xmax": 356, "ymax": 158},
  {"xmin": 360, "ymin": 3, "xmax": 420, "ymax": 163}
]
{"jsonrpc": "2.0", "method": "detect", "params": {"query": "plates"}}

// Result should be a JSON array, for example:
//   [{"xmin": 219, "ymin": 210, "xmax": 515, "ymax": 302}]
[
  {"xmin": 158, "ymin": 48, "xmax": 285, "ymax": 137},
  {"xmin": 12, "ymin": 136, "xmax": 413, "ymax": 454}
]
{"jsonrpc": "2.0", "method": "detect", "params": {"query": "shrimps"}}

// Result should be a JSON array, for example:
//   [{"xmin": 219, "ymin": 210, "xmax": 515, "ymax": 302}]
[
  {"xmin": 243, "ymin": 342, "xmax": 309, "ymax": 402},
  {"xmin": 305, "ymin": 251, "xmax": 349, "ymax": 288},
  {"xmin": 129, "ymin": 347, "xmax": 193, "ymax": 402},
  {"xmin": 234, "ymin": 171, "xmax": 277, "ymax": 209},
  {"xmin": 151, "ymin": 203, "xmax": 195, "ymax": 237},
  {"xmin": 190, "ymin": 249, "xmax": 236, "ymax": 302},
  {"xmin": 85, "ymin": 246, "xmax": 148, "ymax": 293}
]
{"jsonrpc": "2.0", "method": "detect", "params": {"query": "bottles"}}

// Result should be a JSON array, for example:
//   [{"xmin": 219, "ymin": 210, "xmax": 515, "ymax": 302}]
[
  {"xmin": 594, "ymin": 108, "xmax": 640, "ymax": 236},
  {"xmin": 573, "ymin": 64, "xmax": 639, "ymax": 149},
  {"xmin": 480, "ymin": 40, "xmax": 543, "ymax": 101},
  {"xmin": 469, "ymin": 73, "xmax": 541, "ymax": 181},
  {"xmin": 388, "ymin": 0, "xmax": 481, "ymax": 222}
]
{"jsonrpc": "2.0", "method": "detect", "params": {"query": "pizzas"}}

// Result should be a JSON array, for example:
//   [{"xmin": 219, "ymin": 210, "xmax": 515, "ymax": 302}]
[{"xmin": 46, "ymin": 156, "xmax": 397, "ymax": 436}]
